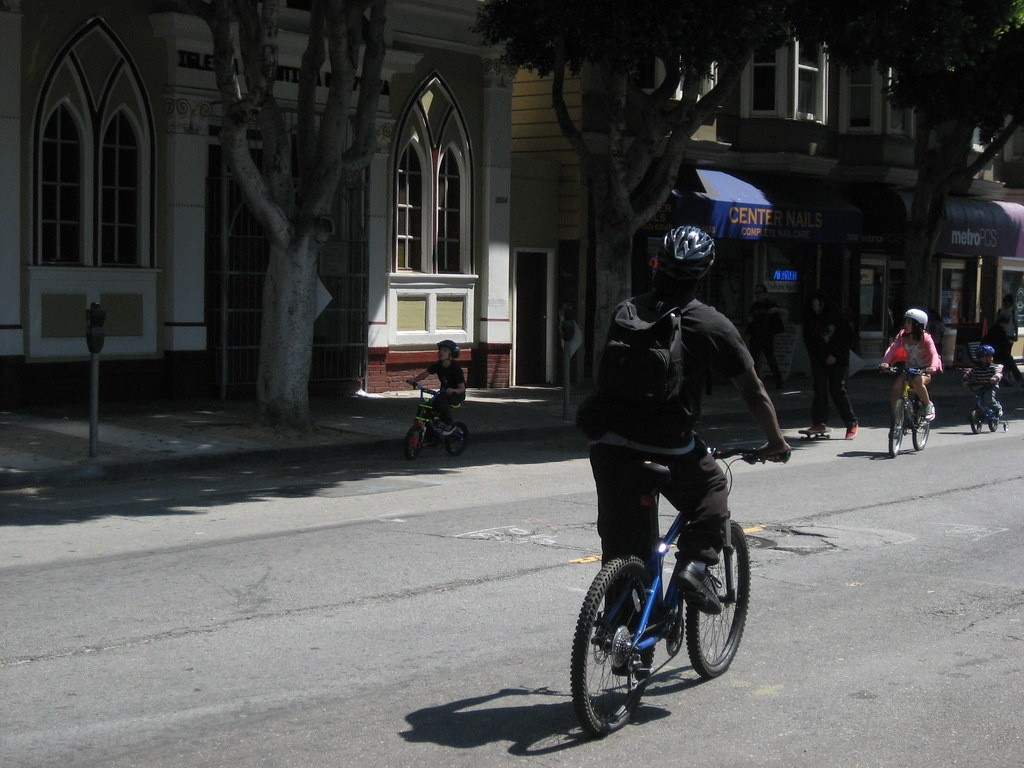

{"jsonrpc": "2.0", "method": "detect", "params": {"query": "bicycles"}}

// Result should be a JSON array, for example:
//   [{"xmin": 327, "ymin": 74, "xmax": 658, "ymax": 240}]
[
  {"xmin": 401, "ymin": 380, "xmax": 469, "ymax": 462},
  {"xmin": 878, "ymin": 363, "xmax": 937, "ymax": 459},
  {"xmin": 962, "ymin": 378, "xmax": 1010, "ymax": 434},
  {"xmin": 568, "ymin": 446, "xmax": 792, "ymax": 735}
]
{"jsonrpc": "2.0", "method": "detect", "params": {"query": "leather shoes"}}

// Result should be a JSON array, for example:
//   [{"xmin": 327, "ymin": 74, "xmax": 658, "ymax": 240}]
[{"xmin": 674, "ymin": 561, "xmax": 721, "ymax": 615}]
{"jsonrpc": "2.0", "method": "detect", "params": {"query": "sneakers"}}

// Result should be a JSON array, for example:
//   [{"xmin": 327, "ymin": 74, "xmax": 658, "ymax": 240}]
[
  {"xmin": 807, "ymin": 424, "xmax": 827, "ymax": 432},
  {"xmin": 924, "ymin": 401, "xmax": 936, "ymax": 422},
  {"xmin": 441, "ymin": 424, "xmax": 457, "ymax": 435},
  {"xmin": 996, "ymin": 405, "xmax": 1003, "ymax": 417},
  {"xmin": 845, "ymin": 422, "xmax": 859, "ymax": 440}
]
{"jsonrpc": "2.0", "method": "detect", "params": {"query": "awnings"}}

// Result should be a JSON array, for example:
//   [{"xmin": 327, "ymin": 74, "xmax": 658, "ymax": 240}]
[
  {"xmin": 933, "ymin": 194, "xmax": 1024, "ymax": 259},
  {"xmin": 635, "ymin": 159, "xmax": 909, "ymax": 244}
]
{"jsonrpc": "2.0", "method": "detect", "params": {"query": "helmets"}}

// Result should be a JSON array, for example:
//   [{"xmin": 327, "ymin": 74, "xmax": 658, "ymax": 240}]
[
  {"xmin": 657, "ymin": 225, "xmax": 716, "ymax": 278},
  {"xmin": 974, "ymin": 345, "xmax": 995, "ymax": 357},
  {"xmin": 905, "ymin": 309, "xmax": 928, "ymax": 330},
  {"xmin": 437, "ymin": 340, "xmax": 461, "ymax": 358}
]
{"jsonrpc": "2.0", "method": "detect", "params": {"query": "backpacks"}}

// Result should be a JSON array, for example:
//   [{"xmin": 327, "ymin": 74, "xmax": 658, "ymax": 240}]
[{"xmin": 592, "ymin": 294, "xmax": 702, "ymax": 447}]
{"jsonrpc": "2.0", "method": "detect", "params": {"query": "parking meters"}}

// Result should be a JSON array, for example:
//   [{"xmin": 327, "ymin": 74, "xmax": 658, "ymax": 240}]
[
  {"xmin": 84, "ymin": 302, "xmax": 107, "ymax": 457},
  {"xmin": 560, "ymin": 303, "xmax": 579, "ymax": 421}
]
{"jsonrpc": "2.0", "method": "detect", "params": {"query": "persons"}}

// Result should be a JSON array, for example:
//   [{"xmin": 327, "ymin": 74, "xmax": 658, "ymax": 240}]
[
  {"xmin": 746, "ymin": 283, "xmax": 784, "ymax": 393},
  {"xmin": 980, "ymin": 294, "xmax": 1024, "ymax": 389},
  {"xmin": 962, "ymin": 344, "xmax": 1004, "ymax": 423},
  {"xmin": 927, "ymin": 310, "xmax": 945, "ymax": 375},
  {"xmin": 801, "ymin": 291, "xmax": 859, "ymax": 438},
  {"xmin": 407, "ymin": 340, "xmax": 466, "ymax": 450},
  {"xmin": 881, "ymin": 308, "xmax": 939, "ymax": 439},
  {"xmin": 584, "ymin": 225, "xmax": 791, "ymax": 676}
]
